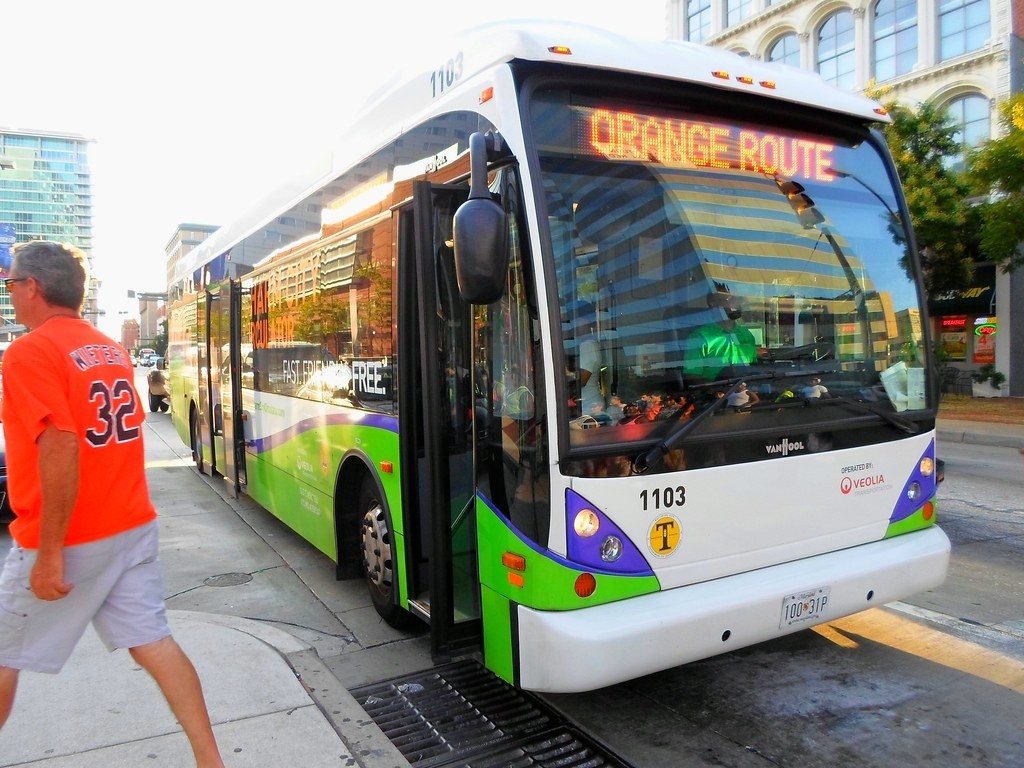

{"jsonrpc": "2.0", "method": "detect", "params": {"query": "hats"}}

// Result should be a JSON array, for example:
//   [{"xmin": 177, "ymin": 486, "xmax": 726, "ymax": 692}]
[{"xmin": 503, "ymin": 365, "xmax": 524, "ymax": 378}]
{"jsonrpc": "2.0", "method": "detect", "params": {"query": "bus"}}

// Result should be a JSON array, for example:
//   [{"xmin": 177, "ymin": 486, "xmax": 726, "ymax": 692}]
[{"xmin": 165, "ymin": 14, "xmax": 954, "ymax": 698}]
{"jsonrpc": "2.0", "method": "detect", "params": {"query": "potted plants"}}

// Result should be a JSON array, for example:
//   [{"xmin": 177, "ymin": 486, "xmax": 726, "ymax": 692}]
[{"xmin": 972, "ymin": 363, "xmax": 1005, "ymax": 399}]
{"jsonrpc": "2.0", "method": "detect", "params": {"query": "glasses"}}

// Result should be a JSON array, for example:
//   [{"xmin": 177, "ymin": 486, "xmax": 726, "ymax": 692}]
[
  {"xmin": 720, "ymin": 296, "xmax": 739, "ymax": 302},
  {"xmin": 3, "ymin": 277, "xmax": 29, "ymax": 293}
]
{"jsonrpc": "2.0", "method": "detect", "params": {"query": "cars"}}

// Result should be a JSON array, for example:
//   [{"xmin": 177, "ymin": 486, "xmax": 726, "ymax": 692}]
[
  {"xmin": 129, "ymin": 348, "xmax": 158, "ymax": 367},
  {"xmin": 147, "ymin": 340, "xmax": 198, "ymax": 413}
]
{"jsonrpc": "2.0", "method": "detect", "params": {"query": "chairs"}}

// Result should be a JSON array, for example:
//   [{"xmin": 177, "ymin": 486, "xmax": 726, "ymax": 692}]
[{"xmin": 941, "ymin": 368, "xmax": 976, "ymax": 395}]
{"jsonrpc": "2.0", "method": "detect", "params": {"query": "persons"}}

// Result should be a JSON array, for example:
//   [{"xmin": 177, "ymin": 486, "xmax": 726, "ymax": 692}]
[
  {"xmin": 578, "ymin": 300, "xmax": 606, "ymax": 417},
  {"xmin": 683, "ymin": 282, "xmax": 760, "ymax": 404},
  {"xmin": 0, "ymin": 240, "xmax": 226, "ymax": 767},
  {"xmin": 446, "ymin": 358, "xmax": 536, "ymax": 423},
  {"xmin": 564, "ymin": 360, "xmax": 831, "ymax": 431}
]
